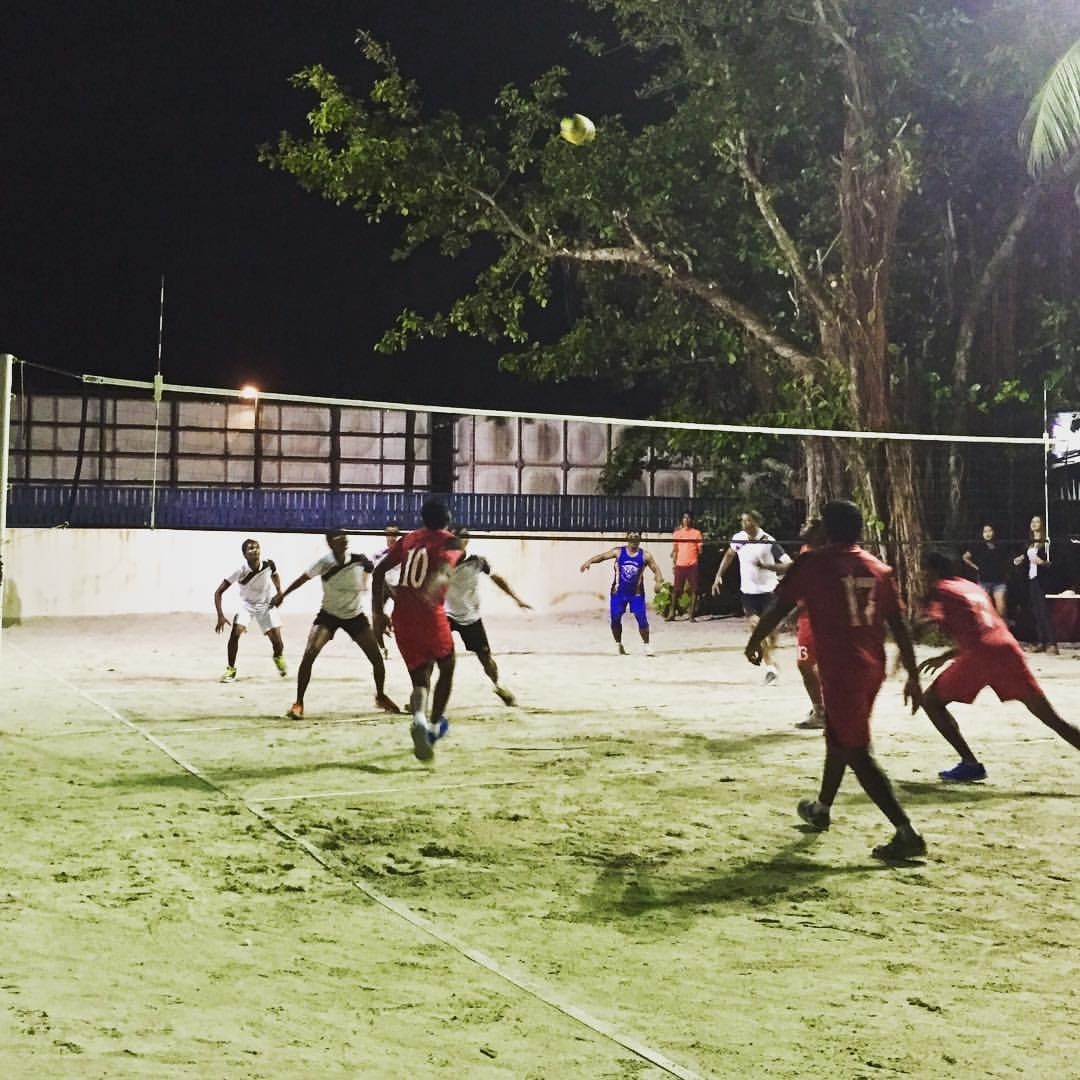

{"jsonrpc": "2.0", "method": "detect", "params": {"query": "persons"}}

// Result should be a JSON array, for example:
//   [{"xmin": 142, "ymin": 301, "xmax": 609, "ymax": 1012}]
[
  {"xmin": 214, "ymin": 539, "xmax": 288, "ymax": 683},
  {"xmin": 372, "ymin": 499, "xmax": 465, "ymax": 761},
  {"xmin": 664, "ymin": 512, "xmax": 704, "ymax": 622},
  {"xmin": 372, "ymin": 525, "xmax": 403, "ymax": 660},
  {"xmin": 794, "ymin": 518, "xmax": 826, "ymax": 729},
  {"xmin": 745, "ymin": 499, "xmax": 927, "ymax": 859},
  {"xmin": 891, "ymin": 552, "xmax": 1080, "ymax": 779},
  {"xmin": 712, "ymin": 509, "xmax": 793, "ymax": 686},
  {"xmin": 581, "ymin": 528, "xmax": 664, "ymax": 656},
  {"xmin": 276, "ymin": 529, "xmax": 400, "ymax": 719},
  {"xmin": 962, "ymin": 522, "xmax": 1009, "ymax": 629},
  {"xmin": 404, "ymin": 525, "xmax": 531, "ymax": 711},
  {"xmin": 1012, "ymin": 514, "xmax": 1059, "ymax": 655}
]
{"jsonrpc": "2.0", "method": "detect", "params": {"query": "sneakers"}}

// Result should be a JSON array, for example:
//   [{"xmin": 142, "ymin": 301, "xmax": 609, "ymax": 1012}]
[
  {"xmin": 643, "ymin": 644, "xmax": 655, "ymax": 655},
  {"xmin": 495, "ymin": 685, "xmax": 515, "ymax": 705},
  {"xmin": 219, "ymin": 664, "xmax": 237, "ymax": 683},
  {"xmin": 872, "ymin": 823, "xmax": 929, "ymax": 858},
  {"xmin": 428, "ymin": 716, "xmax": 448, "ymax": 742},
  {"xmin": 286, "ymin": 702, "xmax": 304, "ymax": 720},
  {"xmin": 793, "ymin": 708, "xmax": 827, "ymax": 728},
  {"xmin": 404, "ymin": 695, "xmax": 429, "ymax": 712},
  {"xmin": 375, "ymin": 694, "xmax": 400, "ymax": 713},
  {"xmin": 938, "ymin": 759, "xmax": 989, "ymax": 780},
  {"xmin": 272, "ymin": 654, "xmax": 287, "ymax": 676},
  {"xmin": 409, "ymin": 719, "xmax": 434, "ymax": 761},
  {"xmin": 616, "ymin": 641, "xmax": 626, "ymax": 654},
  {"xmin": 796, "ymin": 799, "xmax": 831, "ymax": 831}
]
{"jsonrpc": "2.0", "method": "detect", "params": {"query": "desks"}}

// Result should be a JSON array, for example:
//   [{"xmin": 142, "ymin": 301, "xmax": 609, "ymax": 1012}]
[{"xmin": 1045, "ymin": 594, "xmax": 1080, "ymax": 643}]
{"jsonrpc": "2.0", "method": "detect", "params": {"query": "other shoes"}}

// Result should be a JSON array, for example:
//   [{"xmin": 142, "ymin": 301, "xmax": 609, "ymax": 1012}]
[
  {"xmin": 765, "ymin": 662, "xmax": 779, "ymax": 686},
  {"xmin": 1024, "ymin": 642, "xmax": 1058, "ymax": 655},
  {"xmin": 664, "ymin": 616, "xmax": 675, "ymax": 622},
  {"xmin": 688, "ymin": 616, "xmax": 696, "ymax": 622}
]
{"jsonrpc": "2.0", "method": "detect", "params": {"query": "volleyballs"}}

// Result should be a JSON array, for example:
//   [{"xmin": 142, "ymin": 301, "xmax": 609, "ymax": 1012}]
[{"xmin": 560, "ymin": 114, "xmax": 595, "ymax": 146}]
